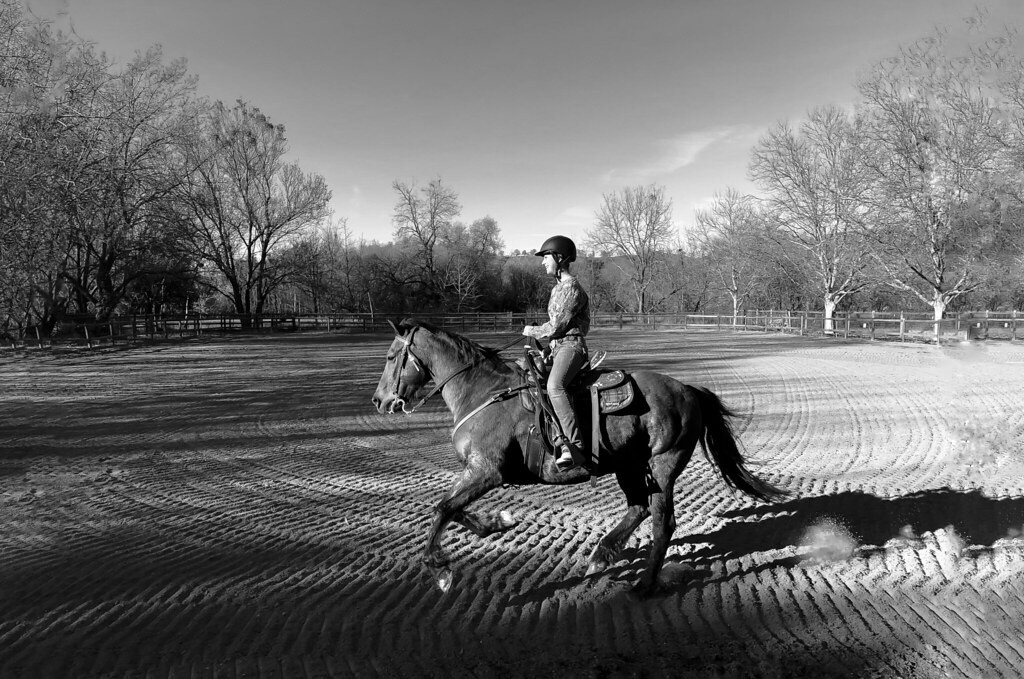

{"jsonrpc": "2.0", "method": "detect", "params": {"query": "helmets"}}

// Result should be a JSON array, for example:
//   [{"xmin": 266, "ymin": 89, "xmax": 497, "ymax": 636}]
[{"xmin": 535, "ymin": 236, "xmax": 576, "ymax": 262}]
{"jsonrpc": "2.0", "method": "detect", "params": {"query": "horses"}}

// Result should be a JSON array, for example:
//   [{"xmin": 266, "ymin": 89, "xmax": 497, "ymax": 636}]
[{"xmin": 371, "ymin": 315, "xmax": 793, "ymax": 613}]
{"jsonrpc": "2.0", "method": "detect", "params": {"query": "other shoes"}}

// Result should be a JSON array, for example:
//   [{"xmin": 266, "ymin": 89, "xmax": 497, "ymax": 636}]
[{"xmin": 555, "ymin": 447, "xmax": 572, "ymax": 465}]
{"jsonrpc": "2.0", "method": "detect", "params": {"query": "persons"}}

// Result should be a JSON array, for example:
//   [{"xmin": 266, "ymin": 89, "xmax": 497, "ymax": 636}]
[{"xmin": 522, "ymin": 234, "xmax": 593, "ymax": 463}]
